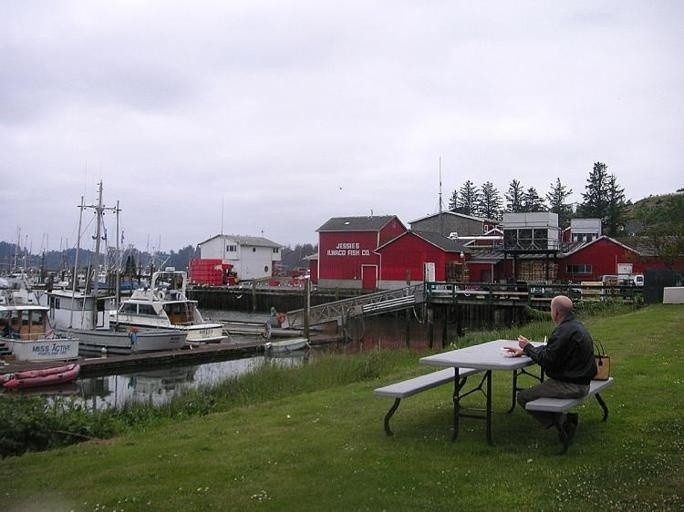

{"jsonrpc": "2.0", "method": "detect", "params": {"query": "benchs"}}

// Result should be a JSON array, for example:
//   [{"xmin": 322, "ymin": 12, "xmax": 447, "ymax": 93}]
[{"xmin": 374, "ymin": 366, "xmax": 615, "ymax": 456}]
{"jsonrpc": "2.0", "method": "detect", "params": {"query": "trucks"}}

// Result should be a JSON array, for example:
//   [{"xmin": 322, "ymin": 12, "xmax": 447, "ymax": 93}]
[
  {"xmin": 188, "ymin": 255, "xmax": 240, "ymax": 287},
  {"xmin": 268, "ymin": 263, "xmax": 308, "ymax": 289}
]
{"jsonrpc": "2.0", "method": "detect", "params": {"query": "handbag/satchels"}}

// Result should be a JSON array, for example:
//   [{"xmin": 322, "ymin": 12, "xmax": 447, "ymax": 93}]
[{"xmin": 592, "ymin": 355, "xmax": 610, "ymax": 380}]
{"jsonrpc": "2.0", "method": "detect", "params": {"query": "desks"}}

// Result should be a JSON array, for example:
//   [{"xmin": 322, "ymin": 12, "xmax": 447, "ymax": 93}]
[{"xmin": 418, "ymin": 338, "xmax": 549, "ymax": 446}]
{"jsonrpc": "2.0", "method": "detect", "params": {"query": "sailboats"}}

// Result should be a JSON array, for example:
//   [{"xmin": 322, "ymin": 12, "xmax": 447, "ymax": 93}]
[{"xmin": 0, "ymin": 178, "xmax": 228, "ymax": 361}]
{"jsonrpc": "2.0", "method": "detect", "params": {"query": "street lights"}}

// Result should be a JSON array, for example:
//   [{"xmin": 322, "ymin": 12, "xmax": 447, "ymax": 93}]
[{"xmin": 459, "ymin": 249, "xmax": 465, "ymax": 289}]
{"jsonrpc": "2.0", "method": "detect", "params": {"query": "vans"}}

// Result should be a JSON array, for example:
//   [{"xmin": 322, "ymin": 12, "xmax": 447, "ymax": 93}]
[{"xmin": 567, "ymin": 273, "xmax": 644, "ymax": 292}]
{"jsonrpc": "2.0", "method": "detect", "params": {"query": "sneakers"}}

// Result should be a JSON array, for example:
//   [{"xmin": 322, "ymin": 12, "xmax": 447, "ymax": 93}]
[{"xmin": 558, "ymin": 413, "xmax": 578, "ymax": 442}]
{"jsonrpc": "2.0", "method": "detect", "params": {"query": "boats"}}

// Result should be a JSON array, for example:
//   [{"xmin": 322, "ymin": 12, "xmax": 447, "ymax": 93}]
[
  {"xmin": 264, "ymin": 337, "xmax": 309, "ymax": 355},
  {"xmin": 1, "ymin": 362, "xmax": 81, "ymax": 391}
]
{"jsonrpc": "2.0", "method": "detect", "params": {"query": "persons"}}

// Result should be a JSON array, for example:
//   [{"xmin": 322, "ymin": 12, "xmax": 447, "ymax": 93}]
[{"xmin": 502, "ymin": 295, "xmax": 598, "ymax": 457}]
